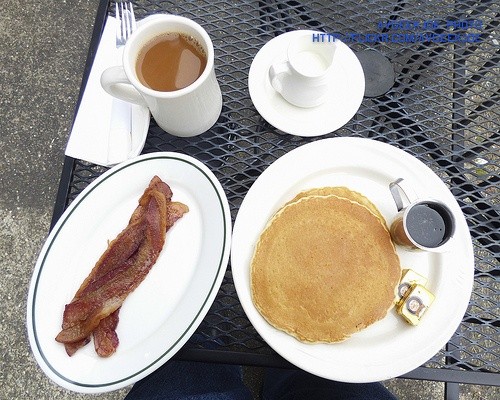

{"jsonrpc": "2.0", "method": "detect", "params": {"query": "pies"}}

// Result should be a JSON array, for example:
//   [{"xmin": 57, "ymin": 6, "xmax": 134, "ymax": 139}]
[{"xmin": 250, "ymin": 185, "xmax": 400, "ymax": 345}]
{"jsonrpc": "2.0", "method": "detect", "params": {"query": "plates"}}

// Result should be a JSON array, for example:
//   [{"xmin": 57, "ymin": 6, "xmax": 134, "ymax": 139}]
[
  {"xmin": 247, "ymin": 29, "xmax": 366, "ymax": 137},
  {"xmin": 230, "ymin": 137, "xmax": 475, "ymax": 384},
  {"xmin": 26, "ymin": 152, "xmax": 232, "ymax": 394}
]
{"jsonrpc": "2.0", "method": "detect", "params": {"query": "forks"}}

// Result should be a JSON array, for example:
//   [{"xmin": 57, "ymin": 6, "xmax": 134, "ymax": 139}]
[{"xmin": 116, "ymin": 1, "xmax": 135, "ymax": 158}]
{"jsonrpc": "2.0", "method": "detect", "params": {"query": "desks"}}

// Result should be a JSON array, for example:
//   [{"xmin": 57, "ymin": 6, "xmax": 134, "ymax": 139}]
[{"xmin": 48, "ymin": 0, "xmax": 500, "ymax": 400}]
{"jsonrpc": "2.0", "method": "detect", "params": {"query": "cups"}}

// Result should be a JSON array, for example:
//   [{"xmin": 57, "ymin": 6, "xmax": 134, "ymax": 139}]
[
  {"xmin": 100, "ymin": 15, "xmax": 223, "ymax": 138},
  {"xmin": 268, "ymin": 34, "xmax": 337, "ymax": 110},
  {"xmin": 389, "ymin": 177, "xmax": 456, "ymax": 252}
]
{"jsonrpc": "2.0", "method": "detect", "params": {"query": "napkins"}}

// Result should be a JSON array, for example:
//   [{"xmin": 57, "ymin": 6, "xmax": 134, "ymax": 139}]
[{"xmin": 64, "ymin": 15, "xmax": 150, "ymax": 168}]
